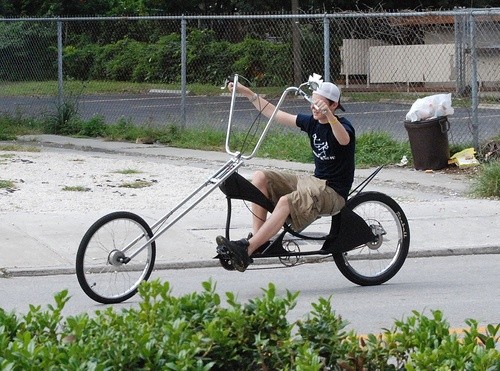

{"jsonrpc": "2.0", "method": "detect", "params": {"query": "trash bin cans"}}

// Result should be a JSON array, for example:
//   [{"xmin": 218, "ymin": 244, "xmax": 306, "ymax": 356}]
[{"xmin": 404, "ymin": 115, "xmax": 450, "ymax": 170}]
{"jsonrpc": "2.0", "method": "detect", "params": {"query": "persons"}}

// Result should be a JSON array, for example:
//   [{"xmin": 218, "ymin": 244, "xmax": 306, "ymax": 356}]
[{"xmin": 216, "ymin": 82, "xmax": 355, "ymax": 273}]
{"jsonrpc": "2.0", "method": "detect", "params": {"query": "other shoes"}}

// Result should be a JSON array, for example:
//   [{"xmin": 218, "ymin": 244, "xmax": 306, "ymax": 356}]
[{"xmin": 216, "ymin": 235, "xmax": 253, "ymax": 272}]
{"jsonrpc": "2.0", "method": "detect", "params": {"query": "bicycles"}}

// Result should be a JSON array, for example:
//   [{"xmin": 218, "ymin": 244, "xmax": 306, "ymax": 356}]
[{"xmin": 75, "ymin": 73, "xmax": 410, "ymax": 304}]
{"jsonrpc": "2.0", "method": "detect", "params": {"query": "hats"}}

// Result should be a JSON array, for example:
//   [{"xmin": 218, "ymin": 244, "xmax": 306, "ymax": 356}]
[{"xmin": 312, "ymin": 82, "xmax": 345, "ymax": 112}]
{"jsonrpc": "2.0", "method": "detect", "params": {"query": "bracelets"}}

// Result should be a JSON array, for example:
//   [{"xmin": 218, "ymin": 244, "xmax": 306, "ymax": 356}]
[
  {"xmin": 248, "ymin": 93, "xmax": 258, "ymax": 103},
  {"xmin": 328, "ymin": 117, "xmax": 338, "ymax": 124}
]
